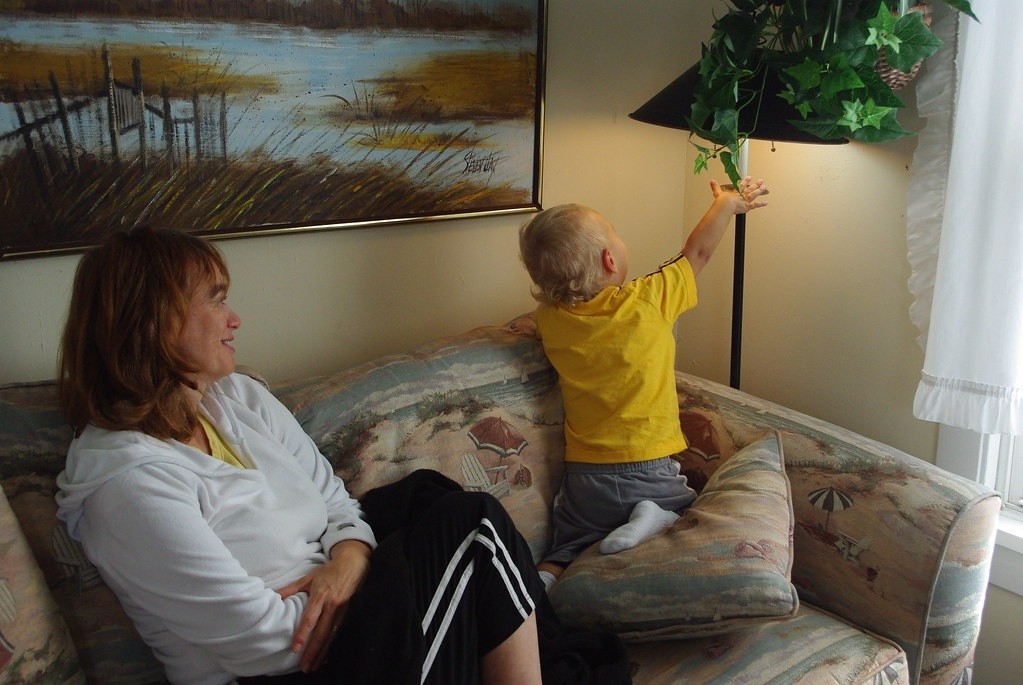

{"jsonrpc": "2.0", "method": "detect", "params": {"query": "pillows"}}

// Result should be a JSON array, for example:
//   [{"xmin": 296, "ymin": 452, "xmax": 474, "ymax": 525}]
[
  {"xmin": 379, "ymin": 388, "xmax": 569, "ymax": 572},
  {"xmin": 542, "ymin": 428, "xmax": 796, "ymax": 645},
  {"xmin": 1, "ymin": 475, "xmax": 181, "ymax": 684}
]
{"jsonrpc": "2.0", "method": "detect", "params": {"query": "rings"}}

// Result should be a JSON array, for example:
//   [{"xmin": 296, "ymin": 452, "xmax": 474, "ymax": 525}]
[{"xmin": 332, "ymin": 626, "xmax": 339, "ymax": 632}]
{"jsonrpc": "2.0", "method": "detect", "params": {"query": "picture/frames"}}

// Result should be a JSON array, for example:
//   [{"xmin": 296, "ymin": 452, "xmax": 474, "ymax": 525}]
[{"xmin": 0, "ymin": 0, "xmax": 548, "ymax": 264}]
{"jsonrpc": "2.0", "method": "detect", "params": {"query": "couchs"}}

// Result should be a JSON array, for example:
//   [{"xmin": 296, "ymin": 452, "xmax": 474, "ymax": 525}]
[{"xmin": 0, "ymin": 333, "xmax": 1003, "ymax": 685}]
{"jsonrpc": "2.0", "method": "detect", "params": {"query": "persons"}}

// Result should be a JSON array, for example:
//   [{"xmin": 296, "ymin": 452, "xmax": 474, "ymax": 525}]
[
  {"xmin": 52, "ymin": 228, "xmax": 560, "ymax": 685},
  {"xmin": 519, "ymin": 175, "xmax": 768, "ymax": 594}
]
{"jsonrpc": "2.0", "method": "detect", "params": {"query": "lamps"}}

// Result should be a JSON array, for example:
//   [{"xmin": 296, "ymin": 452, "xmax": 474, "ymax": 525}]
[{"xmin": 630, "ymin": 48, "xmax": 854, "ymax": 391}]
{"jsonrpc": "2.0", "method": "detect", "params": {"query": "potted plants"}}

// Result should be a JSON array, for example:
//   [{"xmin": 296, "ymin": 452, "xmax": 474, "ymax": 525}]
[{"xmin": 684, "ymin": 1, "xmax": 982, "ymax": 177}]
